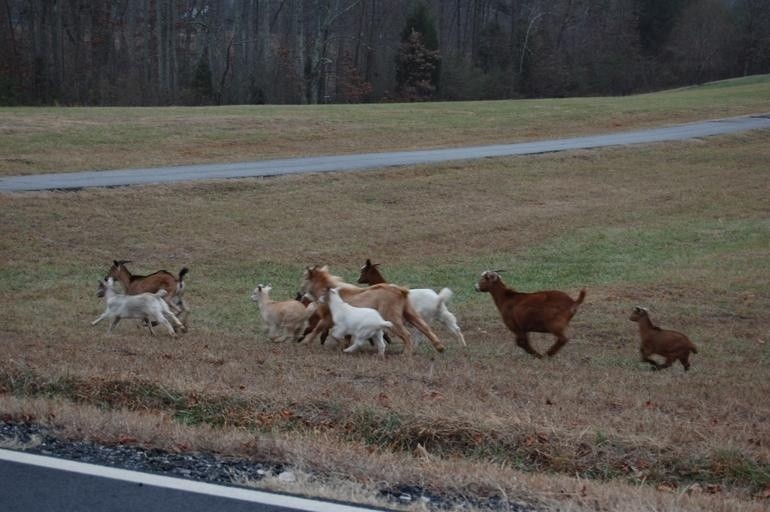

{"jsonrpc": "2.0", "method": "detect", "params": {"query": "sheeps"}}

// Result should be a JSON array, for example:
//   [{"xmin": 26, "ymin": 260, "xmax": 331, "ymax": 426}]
[
  {"xmin": 475, "ymin": 270, "xmax": 587, "ymax": 359},
  {"xmin": 629, "ymin": 306, "xmax": 699, "ymax": 372},
  {"xmin": 294, "ymin": 259, "xmax": 469, "ymax": 361},
  {"xmin": 104, "ymin": 260, "xmax": 190, "ymax": 326},
  {"xmin": 247, "ymin": 283, "xmax": 317, "ymax": 343},
  {"xmin": 91, "ymin": 277, "xmax": 187, "ymax": 339}
]
